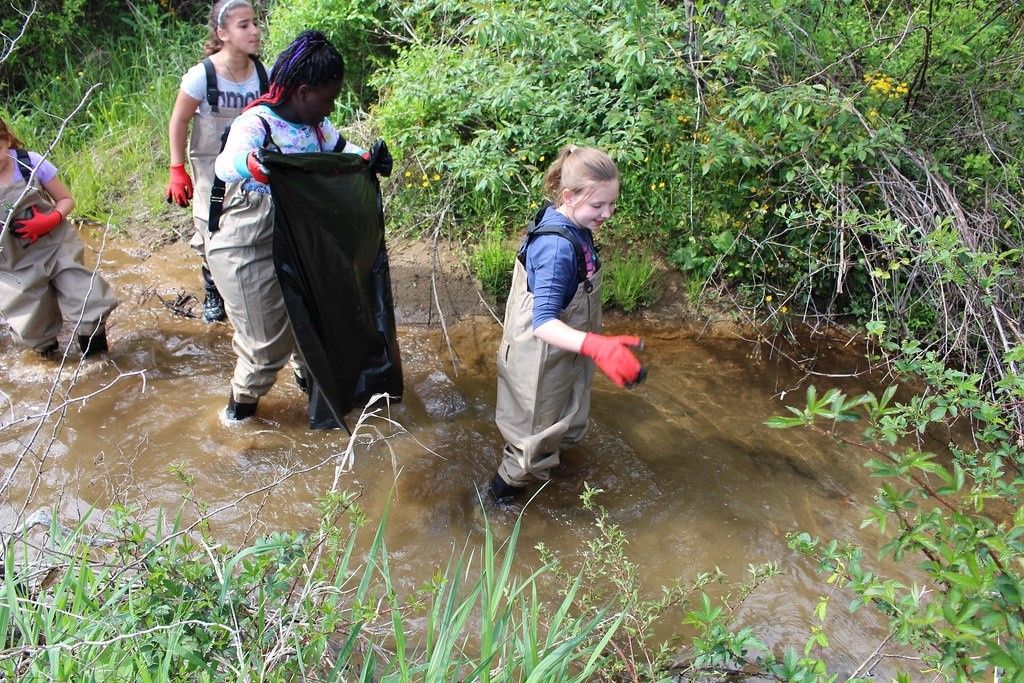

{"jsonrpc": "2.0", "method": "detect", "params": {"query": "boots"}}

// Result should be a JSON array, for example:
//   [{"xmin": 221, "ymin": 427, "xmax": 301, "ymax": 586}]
[
  {"xmin": 38, "ymin": 340, "xmax": 58, "ymax": 358},
  {"xmin": 293, "ymin": 372, "xmax": 311, "ymax": 394},
  {"xmin": 551, "ymin": 466, "xmax": 575, "ymax": 480},
  {"xmin": 487, "ymin": 472, "xmax": 526, "ymax": 506},
  {"xmin": 202, "ymin": 266, "xmax": 226, "ymax": 325},
  {"xmin": 227, "ymin": 388, "xmax": 258, "ymax": 420},
  {"xmin": 77, "ymin": 328, "xmax": 108, "ymax": 359}
]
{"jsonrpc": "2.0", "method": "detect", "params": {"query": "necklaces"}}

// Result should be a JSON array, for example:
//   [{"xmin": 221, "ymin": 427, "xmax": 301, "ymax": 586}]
[{"xmin": 564, "ymin": 205, "xmax": 578, "ymax": 227}]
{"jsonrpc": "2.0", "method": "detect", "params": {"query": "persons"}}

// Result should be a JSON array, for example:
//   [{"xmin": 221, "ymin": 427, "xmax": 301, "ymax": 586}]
[
  {"xmin": 164, "ymin": 0, "xmax": 273, "ymax": 321},
  {"xmin": 0, "ymin": 119, "xmax": 121, "ymax": 360},
  {"xmin": 488, "ymin": 145, "xmax": 647, "ymax": 504},
  {"xmin": 204, "ymin": 32, "xmax": 395, "ymax": 426}
]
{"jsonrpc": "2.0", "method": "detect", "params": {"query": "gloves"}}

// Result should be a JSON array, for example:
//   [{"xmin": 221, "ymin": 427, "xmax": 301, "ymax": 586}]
[
  {"xmin": 9, "ymin": 206, "xmax": 62, "ymax": 249},
  {"xmin": 167, "ymin": 163, "xmax": 193, "ymax": 208},
  {"xmin": 362, "ymin": 138, "xmax": 392, "ymax": 177},
  {"xmin": 579, "ymin": 331, "xmax": 648, "ymax": 390},
  {"xmin": 247, "ymin": 146, "xmax": 270, "ymax": 184}
]
